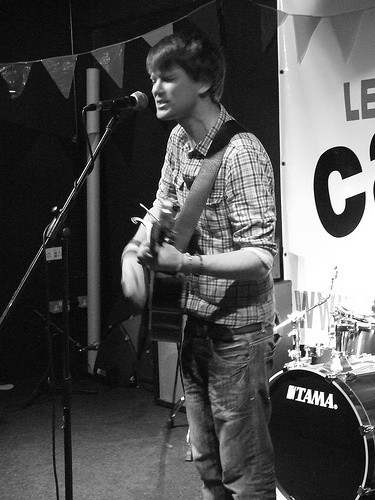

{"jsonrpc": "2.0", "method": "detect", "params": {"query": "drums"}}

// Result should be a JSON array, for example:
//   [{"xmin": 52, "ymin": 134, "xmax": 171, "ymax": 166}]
[
  {"xmin": 332, "ymin": 302, "xmax": 375, "ymax": 355},
  {"xmin": 269, "ymin": 353, "xmax": 375, "ymax": 500}
]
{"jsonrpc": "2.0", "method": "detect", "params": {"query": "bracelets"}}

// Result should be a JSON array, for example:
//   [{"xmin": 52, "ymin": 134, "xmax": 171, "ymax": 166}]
[
  {"xmin": 194, "ymin": 253, "xmax": 203, "ymax": 277},
  {"xmin": 184, "ymin": 252, "xmax": 192, "ymax": 275},
  {"xmin": 130, "ymin": 239, "xmax": 141, "ymax": 246}
]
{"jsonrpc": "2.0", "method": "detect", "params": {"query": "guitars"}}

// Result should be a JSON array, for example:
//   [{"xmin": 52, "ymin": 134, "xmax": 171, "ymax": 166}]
[{"xmin": 140, "ymin": 194, "xmax": 185, "ymax": 342}]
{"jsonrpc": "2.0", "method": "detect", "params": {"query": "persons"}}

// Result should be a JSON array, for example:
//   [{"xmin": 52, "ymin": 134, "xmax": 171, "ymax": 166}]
[{"xmin": 120, "ymin": 32, "xmax": 277, "ymax": 500}]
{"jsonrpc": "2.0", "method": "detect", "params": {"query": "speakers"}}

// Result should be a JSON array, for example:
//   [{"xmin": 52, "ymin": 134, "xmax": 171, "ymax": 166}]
[{"xmin": 152, "ymin": 330, "xmax": 188, "ymax": 412}]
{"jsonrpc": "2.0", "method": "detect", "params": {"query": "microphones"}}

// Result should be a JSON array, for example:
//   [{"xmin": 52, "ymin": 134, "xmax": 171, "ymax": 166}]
[{"xmin": 83, "ymin": 91, "xmax": 148, "ymax": 111}]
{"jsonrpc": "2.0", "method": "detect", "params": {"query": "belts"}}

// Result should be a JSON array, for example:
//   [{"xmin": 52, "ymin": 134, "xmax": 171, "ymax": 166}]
[{"xmin": 189, "ymin": 314, "xmax": 264, "ymax": 340}]
